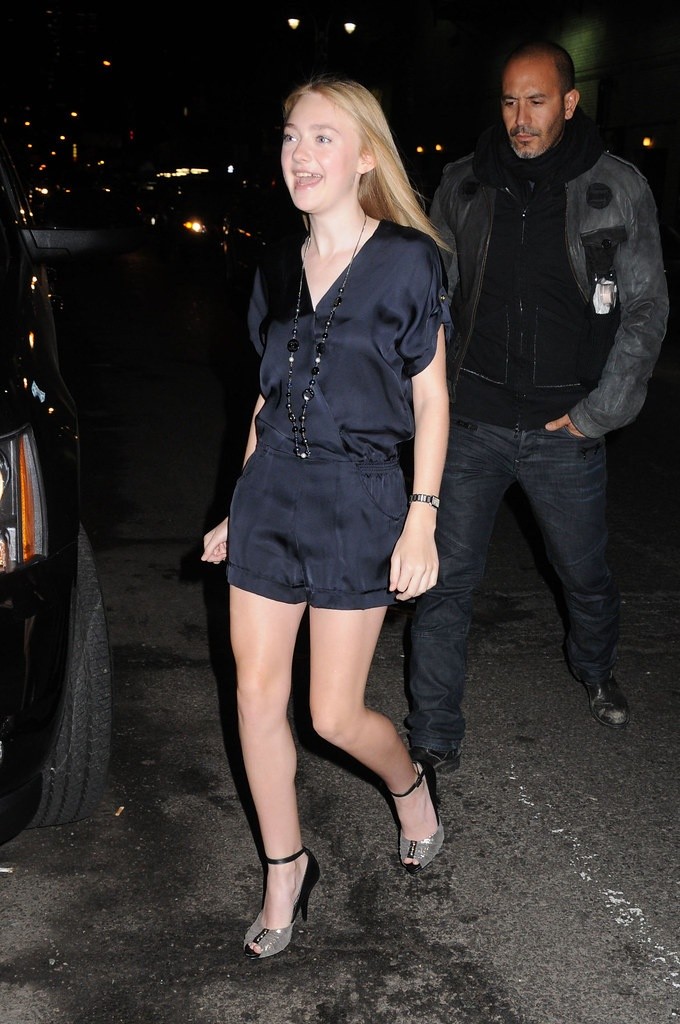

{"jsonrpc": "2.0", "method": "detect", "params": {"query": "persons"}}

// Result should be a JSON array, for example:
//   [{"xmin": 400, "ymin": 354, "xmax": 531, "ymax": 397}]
[
  {"xmin": 409, "ymin": 40, "xmax": 670, "ymax": 773},
  {"xmin": 200, "ymin": 81, "xmax": 455, "ymax": 960}
]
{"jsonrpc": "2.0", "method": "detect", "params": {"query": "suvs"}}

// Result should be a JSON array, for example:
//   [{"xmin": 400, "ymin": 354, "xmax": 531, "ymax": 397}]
[{"xmin": 2, "ymin": 126, "xmax": 110, "ymax": 848}]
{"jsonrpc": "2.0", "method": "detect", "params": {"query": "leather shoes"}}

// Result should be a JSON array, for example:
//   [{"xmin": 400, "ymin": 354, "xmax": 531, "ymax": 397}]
[
  {"xmin": 586, "ymin": 670, "xmax": 629, "ymax": 730},
  {"xmin": 407, "ymin": 744, "xmax": 462, "ymax": 775}
]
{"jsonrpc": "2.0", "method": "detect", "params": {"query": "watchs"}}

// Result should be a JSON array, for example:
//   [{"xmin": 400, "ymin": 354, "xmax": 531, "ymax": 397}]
[{"xmin": 409, "ymin": 494, "xmax": 440, "ymax": 509}]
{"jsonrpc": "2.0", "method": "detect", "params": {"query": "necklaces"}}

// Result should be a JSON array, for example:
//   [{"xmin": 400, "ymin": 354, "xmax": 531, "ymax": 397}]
[{"xmin": 286, "ymin": 214, "xmax": 368, "ymax": 457}]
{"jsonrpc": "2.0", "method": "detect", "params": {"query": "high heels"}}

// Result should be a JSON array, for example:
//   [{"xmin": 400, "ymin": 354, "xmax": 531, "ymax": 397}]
[
  {"xmin": 242, "ymin": 845, "xmax": 321, "ymax": 961},
  {"xmin": 390, "ymin": 759, "xmax": 444, "ymax": 875}
]
